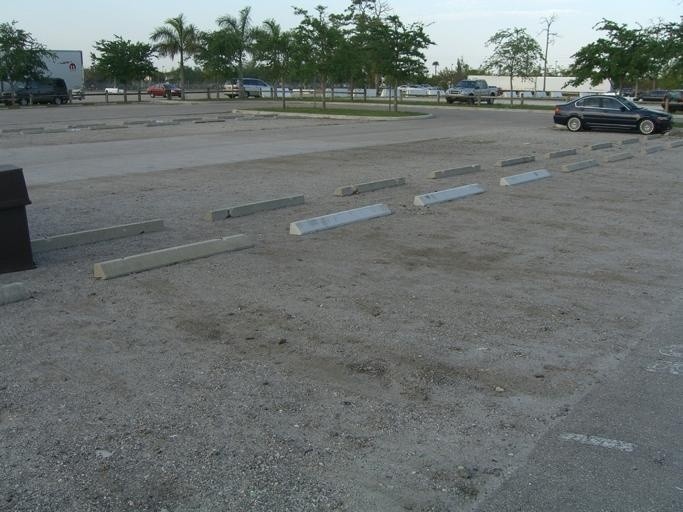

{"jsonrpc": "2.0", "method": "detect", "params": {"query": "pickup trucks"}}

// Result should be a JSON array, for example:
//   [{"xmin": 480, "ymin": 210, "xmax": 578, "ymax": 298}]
[{"xmin": 444, "ymin": 79, "xmax": 497, "ymax": 105}]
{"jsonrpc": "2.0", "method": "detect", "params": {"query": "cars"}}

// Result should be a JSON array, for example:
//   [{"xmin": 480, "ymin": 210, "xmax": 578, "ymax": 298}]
[
  {"xmin": 148, "ymin": 83, "xmax": 182, "ymax": 98},
  {"xmin": 398, "ymin": 83, "xmax": 445, "ymax": 96},
  {"xmin": 622, "ymin": 87, "xmax": 683, "ymax": 113},
  {"xmin": 554, "ymin": 95, "xmax": 673, "ymax": 135},
  {"xmin": 105, "ymin": 85, "xmax": 125, "ymax": 95}
]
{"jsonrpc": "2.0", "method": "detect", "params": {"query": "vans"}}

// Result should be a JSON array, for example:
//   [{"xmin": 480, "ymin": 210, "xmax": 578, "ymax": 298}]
[
  {"xmin": 224, "ymin": 77, "xmax": 275, "ymax": 100},
  {"xmin": 0, "ymin": 77, "xmax": 69, "ymax": 106}
]
